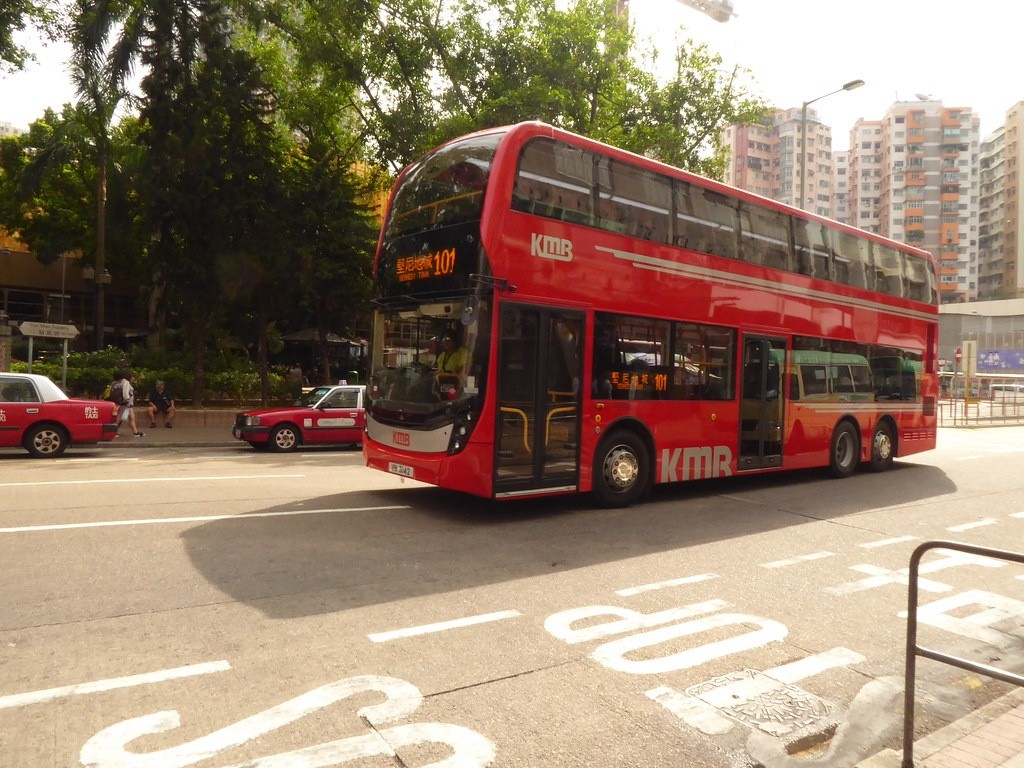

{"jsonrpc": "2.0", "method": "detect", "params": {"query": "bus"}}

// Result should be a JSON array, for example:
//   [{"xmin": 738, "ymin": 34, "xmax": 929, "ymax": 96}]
[
  {"xmin": 739, "ymin": 347, "xmax": 919, "ymax": 450},
  {"xmin": 344, "ymin": 119, "xmax": 938, "ymax": 509}
]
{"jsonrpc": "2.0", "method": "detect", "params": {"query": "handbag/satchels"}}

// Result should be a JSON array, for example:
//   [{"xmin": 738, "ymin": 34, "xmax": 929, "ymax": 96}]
[{"xmin": 107, "ymin": 382, "xmax": 132, "ymax": 406}]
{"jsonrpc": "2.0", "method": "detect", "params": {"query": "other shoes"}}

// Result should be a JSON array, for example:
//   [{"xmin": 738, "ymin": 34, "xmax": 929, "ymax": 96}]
[
  {"xmin": 150, "ymin": 422, "xmax": 156, "ymax": 428},
  {"xmin": 115, "ymin": 433, "xmax": 120, "ymax": 437},
  {"xmin": 165, "ymin": 423, "xmax": 173, "ymax": 428},
  {"xmin": 133, "ymin": 432, "xmax": 146, "ymax": 437}
]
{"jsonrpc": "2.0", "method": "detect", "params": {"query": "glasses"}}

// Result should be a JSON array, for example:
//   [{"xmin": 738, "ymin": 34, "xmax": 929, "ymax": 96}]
[{"xmin": 442, "ymin": 337, "xmax": 451, "ymax": 341}]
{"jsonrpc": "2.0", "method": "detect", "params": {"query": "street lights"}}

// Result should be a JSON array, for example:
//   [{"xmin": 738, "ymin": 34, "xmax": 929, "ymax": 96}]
[{"xmin": 799, "ymin": 80, "xmax": 867, "ymax": 211}]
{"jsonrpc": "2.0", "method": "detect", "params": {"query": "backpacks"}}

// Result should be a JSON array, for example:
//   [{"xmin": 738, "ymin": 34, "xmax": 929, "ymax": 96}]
[{"xmin": 104, "ymin": 381, "xmax": 116, "ymax": 398}]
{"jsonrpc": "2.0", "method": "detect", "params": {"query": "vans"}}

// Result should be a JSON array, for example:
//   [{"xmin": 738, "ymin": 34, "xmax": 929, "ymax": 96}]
[
  {"xmin": 618, "ymin": 337, "xmax": 699, "ymax": 371},
  {"xmin": 989, "ymin": 384, "xmax": 1024, "ymax": 404}
]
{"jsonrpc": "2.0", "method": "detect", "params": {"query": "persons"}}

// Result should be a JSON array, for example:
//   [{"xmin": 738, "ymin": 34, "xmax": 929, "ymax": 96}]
[
  {"xmin": 147, "ymin": 380, "xmax": 176, "ymax": 428},
  {"xmin": 103, "ymin": 371, "xmax": 146, "ymax": 438},
  {"xmin": 432, "ymin": 329, "xmax": 470, "ymax": 384}
]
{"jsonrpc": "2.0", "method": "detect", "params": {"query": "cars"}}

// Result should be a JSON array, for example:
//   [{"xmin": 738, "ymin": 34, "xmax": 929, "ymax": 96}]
[
  {"xmin": 0, "ymin": 373, "xmax": 120, "ymax": 459},
  {"xmin": 231, "ymin": 385, "xmax": 378, "ymax": 452}
]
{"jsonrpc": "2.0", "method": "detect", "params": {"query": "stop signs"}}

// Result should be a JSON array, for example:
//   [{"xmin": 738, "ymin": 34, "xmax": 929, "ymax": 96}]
[{"xmin": 956, "ymin": 348, "xmax": 961, "ymax": 365}]
